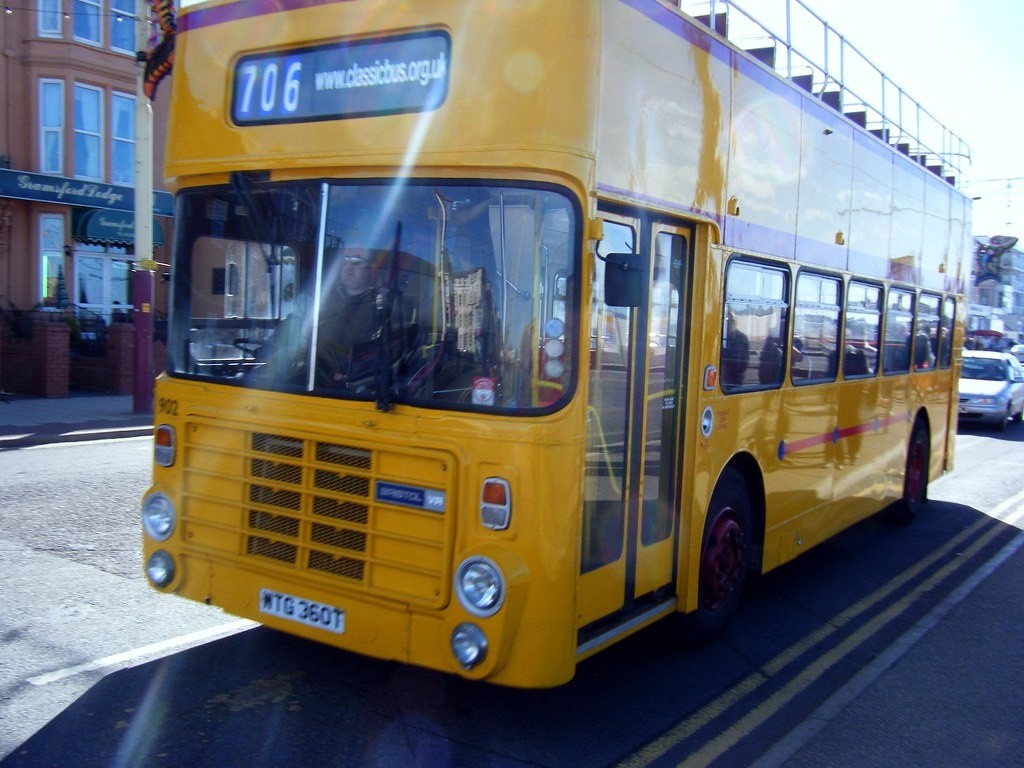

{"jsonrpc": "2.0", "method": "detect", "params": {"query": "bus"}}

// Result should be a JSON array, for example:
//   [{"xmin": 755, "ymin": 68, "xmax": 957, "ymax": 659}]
[{"xmin": 139, "ymin": 1, "xmax": 979, "ymax": 691}]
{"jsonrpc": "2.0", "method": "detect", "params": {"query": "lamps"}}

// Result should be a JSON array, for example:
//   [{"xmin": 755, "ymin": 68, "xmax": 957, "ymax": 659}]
[{"xmin": 64, "ymin": 245, "xmax": 72, "ymax": 256}]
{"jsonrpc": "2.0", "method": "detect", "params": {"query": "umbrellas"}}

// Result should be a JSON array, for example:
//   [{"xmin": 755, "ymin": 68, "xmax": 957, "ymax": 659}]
[{"xmin": 968, "ymin": 329, "xmax": 1003, "ymax": 337}]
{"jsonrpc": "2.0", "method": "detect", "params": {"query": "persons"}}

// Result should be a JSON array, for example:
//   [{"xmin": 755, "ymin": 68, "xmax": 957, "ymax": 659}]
[
  {"xmin": 892, "ymin": 325, "xmax": 954, "ymax": 371},
  {"xmin": 252, "ymin": 229, "xmax": 407, "ymax": 385},
  {"xmin": 723, "ymin": 313, "xmax": 751, "ymax": 390},
  {"xmin": 829, "ymin": 327, "xmax": 877, "ymax": 377},
  {"xmin": 963, "ymin": 335, "xmax": 1024, "ymax": 353},
  {"xmin": 759, "ymin": 321, "xmax": 805, "ymax": 383}
]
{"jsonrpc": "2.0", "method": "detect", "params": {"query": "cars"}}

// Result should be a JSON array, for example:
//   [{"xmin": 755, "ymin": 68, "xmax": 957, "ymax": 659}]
[
  {"xmin": 958, "ymin": 350, "xmax": 1024, "ymax": 432},
  {"xmin": 1010, "ymin": 344, "xmax": 1024, "ymax": 364}
]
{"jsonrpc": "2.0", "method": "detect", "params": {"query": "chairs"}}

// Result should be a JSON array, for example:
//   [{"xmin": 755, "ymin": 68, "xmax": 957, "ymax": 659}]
[
  {"xmin": 538, "ymin": 335, "xmax": 935, "ymax": 407},
  {"xmin": 669, "ymin": 0, "xmax": 955, "ymax": 187}
]
